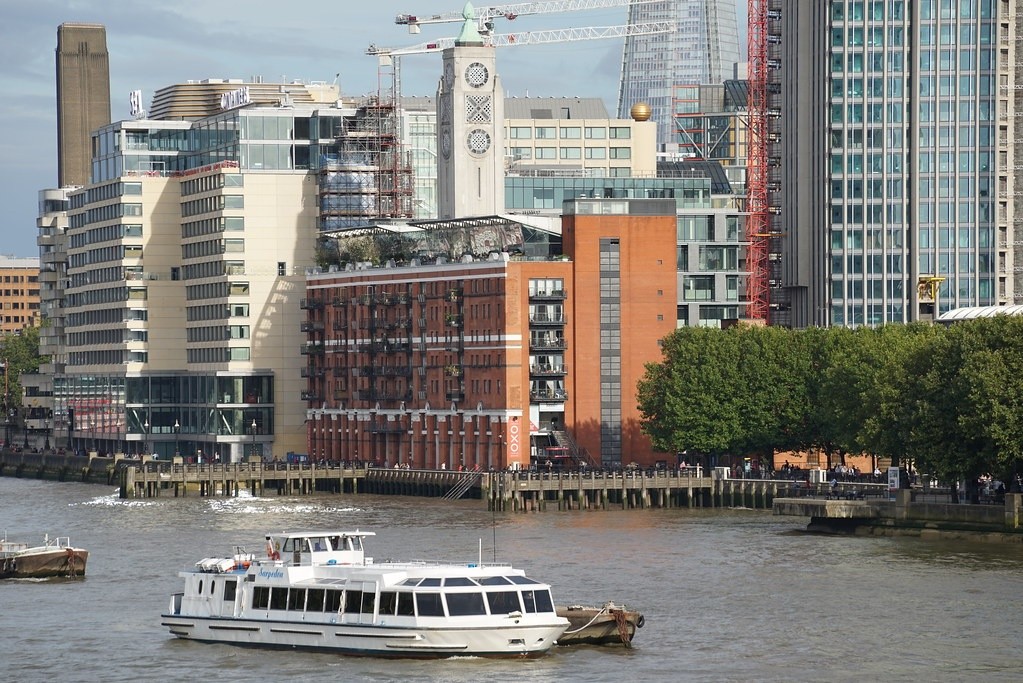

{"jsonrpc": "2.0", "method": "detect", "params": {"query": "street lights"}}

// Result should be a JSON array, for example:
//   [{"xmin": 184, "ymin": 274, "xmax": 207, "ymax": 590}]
[
  {"xmin": 44, "ymin": 418, "xmax": 51, "ymax": 450},
  {"xmin": 144, "ymin": 419, "xmax": 151, "ymax": 455},
  {"xmin": 116, "ymin": 418, "xmax": 122, "ymax": 454},
  {"xmin": 90, "ymin": 420, "xmax": 97, "ymax": 452},
  {"xmin": 24, "ymin": 413, "xmax": 29, "ymax": 448},
  {"xmin": 174, "ymin": 419, "xmax": 181, "ymax": 457},
  {"xmin": 249, "ymin": 419, "xmax": 259, "ymax": 456},
  {"xmin": 66, "ymin": 417, "xmax": 72, "ymax": 451},
  {"xmin": 4, "ymin": 415, "xmax": 10, "ymax": 448}
]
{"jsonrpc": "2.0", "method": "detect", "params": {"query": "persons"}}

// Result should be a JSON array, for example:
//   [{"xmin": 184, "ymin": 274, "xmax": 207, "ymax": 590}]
[
  {"xmin": 1, "ymin": 443, "xmax": 163, "ymax": 460},
  {"xmin": 507, "ymin": 464, "xmax": 513, "ymax": 471},
  {"xmin": 319, "ymin": 455, "xmax": 327, "ymax": 466},
  {"xmin": 441, "ymin": 461, "xmax": 447, "ymax": 471},
  {"xmin": 502, "ymin": 464, "xmax": 508, "ymax": 470},
  {"xmin": 679, "ymin": 460, "xmax": 688, "ymax": 472},
  {"xmin": 186, "ymin": 449, "xmax": 221, "ymax": 465},
  {"xmin": 393, "ymin": 461, "xmax": 412, "ymax": 470},
  {"xmin": 730, "ymin": 461, "xmax": 776, "ymax": 480},
  {"xmin": 780, "ymin": 459, "xmax": 882, "ymax": 496},
  {"xmin": 488, "ymin": 464, "xmax": 494, "ymax": 472},
  {"xmin": 240, "ymin": 454, "xmax": 285, "ymax": 464},
  {"xmin": 293, "ymin": 456, "xmax": 300, "ymax": 465},
  {"xmin": 457, "ymin": 463, "xmax": 469, "ymax": 471}
]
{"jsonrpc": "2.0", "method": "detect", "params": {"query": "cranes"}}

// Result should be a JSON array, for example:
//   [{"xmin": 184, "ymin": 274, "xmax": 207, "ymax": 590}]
[
  {"xmin": 394, "ymin": 0, "xmax": 685, "ymax": 34},
  {"xmin": 364, "ymin": 18, "xmax": 678, "ymax": 64},
  {"xmin": 746, "ymin": 0, "xmax": 771, "ymax": 330}
]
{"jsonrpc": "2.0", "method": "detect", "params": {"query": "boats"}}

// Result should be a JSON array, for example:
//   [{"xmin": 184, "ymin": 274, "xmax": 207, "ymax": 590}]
[
  {"xmin": 0, "ymin": 526, "xmax": 90, "ymax": 582},
  {"xmin": 554, "ymin": 599, "xmax": 644, "ymax": 649},
  {"xmin": 158, "ymin": 528, "xmax": 572, "ymax": 662}
]
